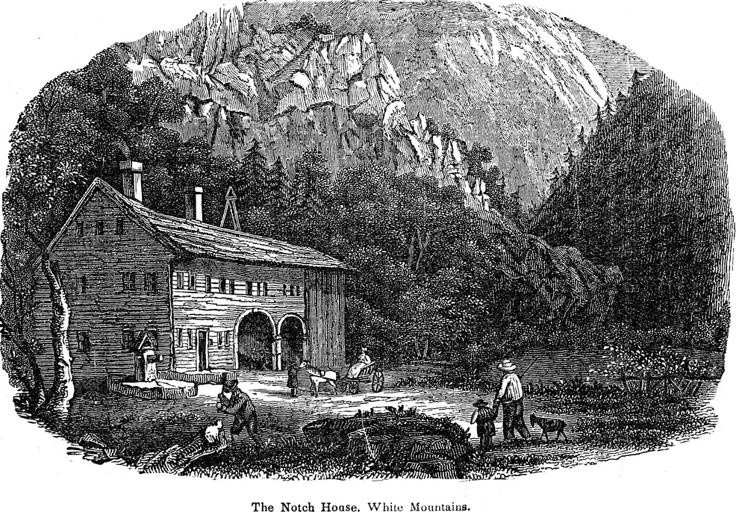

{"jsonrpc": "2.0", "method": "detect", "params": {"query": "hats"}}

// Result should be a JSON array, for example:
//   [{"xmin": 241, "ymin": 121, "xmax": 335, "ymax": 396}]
[
  {"xmin": 473, "ymin": 399, "xmax": 488, "ymax": 407},
  {"xmin": 498, "ymin": 359, "xmax": 517, "ymax": 372},
  {"xmin": 221, "ymin": 372, "xmax": 237, "ymax": 381},
  {"xmin": 362, "ymin": 347, "xmax": 367, "ymax": 351}
]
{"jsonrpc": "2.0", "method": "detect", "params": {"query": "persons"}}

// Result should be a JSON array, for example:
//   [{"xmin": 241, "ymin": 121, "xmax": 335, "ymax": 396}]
[
  {"xmin": 470, "ymin": 400, "xmax": 500, "ymax": 450},
  {"xmin": 494, "ymin": 360, "xmax": 533, "ymax": 442},
  {"xmin": 347, "ymin": 347, "xmax": 371, "ymax": 378},
  {"xmin": 288, "ymin": 362, "xmax": 298, "ymax": 399},
  {"xmin": 216, "ymin": 378, "xmax": 264, "ymax": 453}
]
